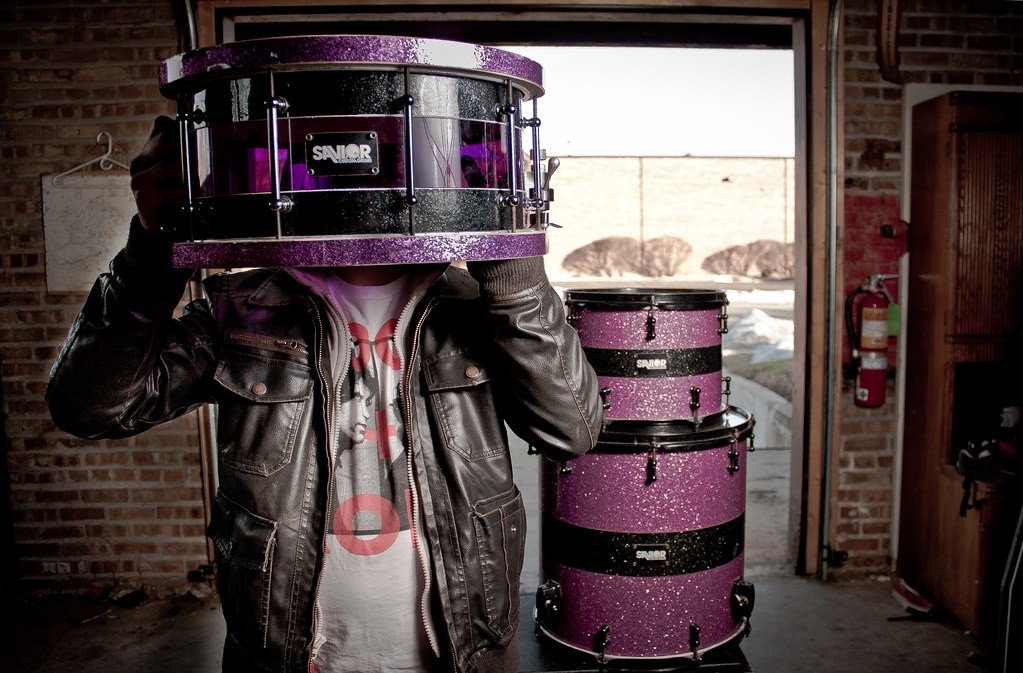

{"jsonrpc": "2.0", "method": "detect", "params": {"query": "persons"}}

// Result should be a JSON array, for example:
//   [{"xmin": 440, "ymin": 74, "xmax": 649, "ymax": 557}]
[{"xmin": 45, "ymin": 116, "xmax": 603, "ymax": 673}]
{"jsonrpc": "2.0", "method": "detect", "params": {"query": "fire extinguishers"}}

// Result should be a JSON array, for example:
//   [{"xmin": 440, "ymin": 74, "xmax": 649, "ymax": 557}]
[{"xmin": 850, "ymin": 271, "xmax": 899, "ymax": 409}]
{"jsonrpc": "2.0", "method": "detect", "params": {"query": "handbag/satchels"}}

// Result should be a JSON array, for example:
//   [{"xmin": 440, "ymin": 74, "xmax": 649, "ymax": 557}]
[{"xmin": 953, "ymin": 438, "xmax": 997, "ymax": 518}]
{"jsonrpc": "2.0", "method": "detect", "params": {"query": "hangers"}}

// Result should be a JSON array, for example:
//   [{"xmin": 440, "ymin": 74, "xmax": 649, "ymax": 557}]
[{"xmin": 52, "ymin": 132, "xmax": 131, "ymax": 192}]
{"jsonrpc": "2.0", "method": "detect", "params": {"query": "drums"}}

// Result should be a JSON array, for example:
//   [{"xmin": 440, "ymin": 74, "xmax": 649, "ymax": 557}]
[
  {"xmin": 530, "ymin": 281, "xmax": 757, "ymax": 672},
  {"xmin": 154, "ymin": 29, "xmax": 564, "ymax": 272}
]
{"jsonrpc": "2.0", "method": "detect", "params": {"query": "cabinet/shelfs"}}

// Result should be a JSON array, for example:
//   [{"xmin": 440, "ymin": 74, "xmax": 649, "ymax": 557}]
[{"xmin": 891, "ymin": 88, "xmax": 1023, "ymax": 673}]
